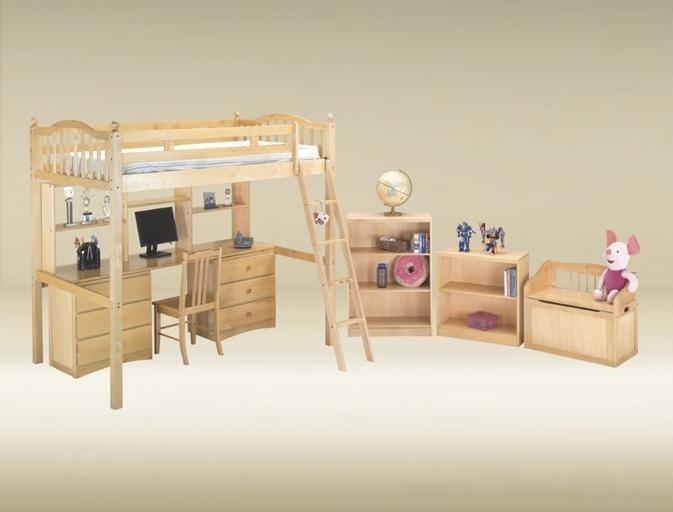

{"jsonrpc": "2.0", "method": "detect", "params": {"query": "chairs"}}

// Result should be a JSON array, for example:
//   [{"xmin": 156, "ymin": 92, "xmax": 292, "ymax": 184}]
[{"xmin": 152, "ymin": 246, "xmax": 225, "ymax": 366}]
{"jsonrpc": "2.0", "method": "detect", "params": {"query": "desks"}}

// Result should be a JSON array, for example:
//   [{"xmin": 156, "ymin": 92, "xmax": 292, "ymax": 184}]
[{"xmin": 47, "ymin": 237, "xmax": 276, "ymax": 379}]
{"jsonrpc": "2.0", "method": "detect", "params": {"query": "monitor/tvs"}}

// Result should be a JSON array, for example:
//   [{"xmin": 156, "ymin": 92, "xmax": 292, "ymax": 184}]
[{"xmin": 135, "ymin": 207, "xmax": 178, "ymax": 259}]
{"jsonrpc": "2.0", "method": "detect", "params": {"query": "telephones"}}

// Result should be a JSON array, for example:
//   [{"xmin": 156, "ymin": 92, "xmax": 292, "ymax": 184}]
[{"xmin": 234, "ymin": 230, "xmax": 254, "ymax": 248}]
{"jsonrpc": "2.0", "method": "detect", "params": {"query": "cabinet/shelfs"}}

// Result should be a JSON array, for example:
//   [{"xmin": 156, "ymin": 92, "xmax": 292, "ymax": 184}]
[{"xmin": 346, "ymin": 213, "xmax": 528, "ymax": 347}]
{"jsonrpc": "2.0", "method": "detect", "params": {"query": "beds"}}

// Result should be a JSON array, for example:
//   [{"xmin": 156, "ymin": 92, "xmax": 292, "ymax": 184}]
[{"xmin": 29, "ymin": 110, "xmax": 337, "ymax": 410}]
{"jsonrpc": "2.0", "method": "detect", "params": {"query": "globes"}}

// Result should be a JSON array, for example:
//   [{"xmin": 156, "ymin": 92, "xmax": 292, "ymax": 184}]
[{"xmin": 376, "ymin": 169, "xmax": 412, "ymax": 216}]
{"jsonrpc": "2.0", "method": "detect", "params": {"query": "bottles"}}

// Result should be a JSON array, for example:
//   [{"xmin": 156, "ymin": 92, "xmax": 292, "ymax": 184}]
[{"xmin": 376, "ymin": 263, "xmax": 387, "ymax": 288}]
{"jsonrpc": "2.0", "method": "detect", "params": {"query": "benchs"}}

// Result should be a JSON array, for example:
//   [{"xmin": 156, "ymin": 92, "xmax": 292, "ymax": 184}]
[{"xmin": 523, "ymin": 257, "xmax": 638, "ymax": 368}]
